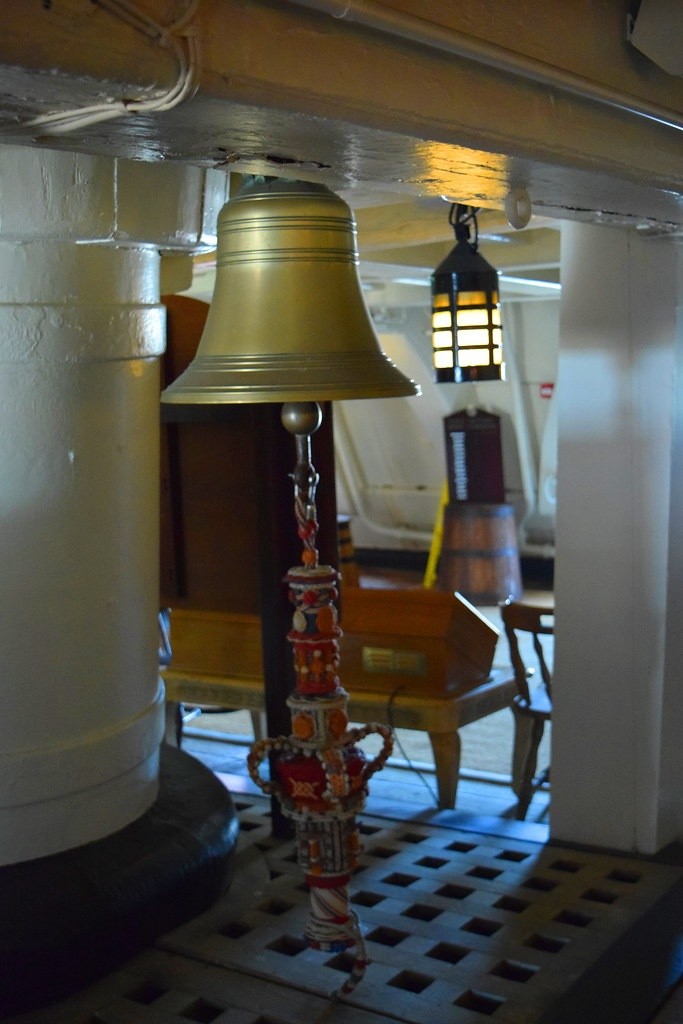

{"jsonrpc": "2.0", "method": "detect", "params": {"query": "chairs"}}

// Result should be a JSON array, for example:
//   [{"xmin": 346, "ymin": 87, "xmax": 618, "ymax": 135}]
[{"xmin": 498, "ymin": 597, "xmax": 554, "ymax": 821}]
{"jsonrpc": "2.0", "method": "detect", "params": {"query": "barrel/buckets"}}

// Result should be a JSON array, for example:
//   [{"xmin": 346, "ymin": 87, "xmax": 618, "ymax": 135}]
[
  {"xmin": 441, "ymin": 502, "xmax": 521, "ymax": 606},
  {"xmin": 336, "ymin": 514, "xmax": 358, "ymax": 588}
]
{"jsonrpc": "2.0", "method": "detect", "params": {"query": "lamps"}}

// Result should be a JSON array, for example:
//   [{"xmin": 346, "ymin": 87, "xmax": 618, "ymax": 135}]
[{"xmin": 429, "ymin": 205, "xmax": 506, "ymax": 384}]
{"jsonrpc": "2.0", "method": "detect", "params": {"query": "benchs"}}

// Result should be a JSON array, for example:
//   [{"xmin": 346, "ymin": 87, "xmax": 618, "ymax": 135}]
[{"xmin": 162, "ymin": 586, "xmax": 536, "ymax": 810}]
